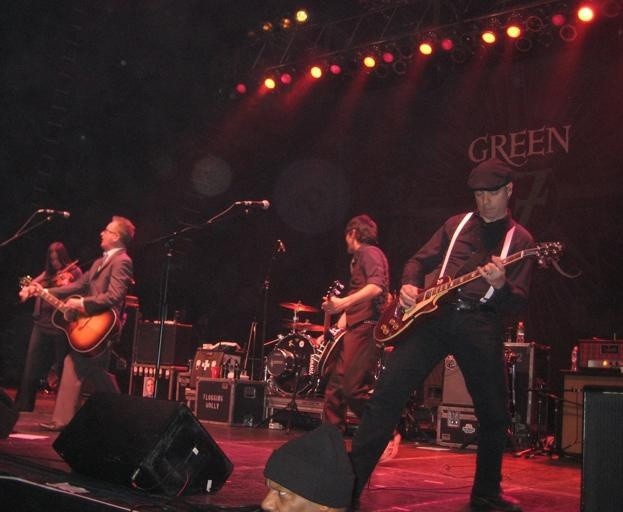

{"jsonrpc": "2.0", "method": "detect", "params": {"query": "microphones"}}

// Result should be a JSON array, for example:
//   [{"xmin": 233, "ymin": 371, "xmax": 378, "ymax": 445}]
[
  {"xmin": 237, "ymin": 199, "xmax": 269, "ymax": 211},
  {"xmin": 278, "ymin": 237, "xmax": 287, "ymax": 256},
  {"xmin": 39, "ymin": 209, "xmax": 70, "ymax": 219}
]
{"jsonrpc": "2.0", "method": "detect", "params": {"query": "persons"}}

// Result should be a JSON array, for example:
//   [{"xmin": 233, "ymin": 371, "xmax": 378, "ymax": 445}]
[
  {"xmin": 348, "ymin": 157, "xmax": 538, "ymax": 510},
  {"xmin": 322, "ymin": 214, "xmax": 401, "ymax": 465},
  {"xmin": 14, "ymin": 242, "xmax": 86, "ymax": 415},
  {"xmin": 29, "ymin": 215, "xmax": 135, "ymax": 433}
]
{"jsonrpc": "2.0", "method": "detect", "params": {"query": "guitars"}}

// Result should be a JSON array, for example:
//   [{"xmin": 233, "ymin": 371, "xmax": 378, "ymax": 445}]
[
  {"xmin": 18, "ymin": 273, "xmax": 120, "ymax": 359},
  {"xmin": 373, "ymin": 241, "xmax": 563, "ymax": 346}
]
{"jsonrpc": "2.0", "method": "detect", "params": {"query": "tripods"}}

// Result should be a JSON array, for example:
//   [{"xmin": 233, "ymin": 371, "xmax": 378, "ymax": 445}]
[{"xmin": 253, "ymin": 354, "xmax": 312, "ymax": 433}]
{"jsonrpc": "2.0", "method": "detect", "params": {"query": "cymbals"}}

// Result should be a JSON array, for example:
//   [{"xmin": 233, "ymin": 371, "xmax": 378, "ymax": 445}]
[
  {"xmin": 287, "ymin": 323, "xmax": 324, "ymax": 332},
  {"xmin": 282, "ymin": 303, "xmax": 318, "ymax": 313}
]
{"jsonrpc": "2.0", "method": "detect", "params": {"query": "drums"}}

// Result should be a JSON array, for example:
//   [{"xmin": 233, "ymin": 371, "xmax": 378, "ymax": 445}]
[
  {"xmin": 267, "ymin": 332, "xmax": 320, "ymax": 394},
  {"xmin": 266, "ymin": 349, "xmax": 294, "ymax": 377}
]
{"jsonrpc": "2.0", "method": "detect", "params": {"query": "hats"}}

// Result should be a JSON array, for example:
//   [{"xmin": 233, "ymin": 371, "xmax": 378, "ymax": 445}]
[{"xmin": 467, "ymin": 159, "xmax": 512, "ymax": 191}]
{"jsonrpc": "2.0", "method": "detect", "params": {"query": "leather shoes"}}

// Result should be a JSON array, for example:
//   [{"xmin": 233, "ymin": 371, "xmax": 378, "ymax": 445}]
[
  {"xmin": 469, "ymin": 493, "xmax": 520, "ymax": 511},
  {"xmin": 39, "ymin": 423, "xmax": 63, "ymax": 430}
]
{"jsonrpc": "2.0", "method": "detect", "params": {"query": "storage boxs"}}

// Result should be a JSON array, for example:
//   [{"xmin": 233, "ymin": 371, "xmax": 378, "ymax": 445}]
[
  {"xmin": 504, "ymin": 342, "xmax": 551, "ymax": 434},
  {"xmin": 435, "ymin": 406, "xmax": 480, "ymax": 451},
  {"xmin": 195, "ymin": 376, "xmax": 266, "ymax": 427}
]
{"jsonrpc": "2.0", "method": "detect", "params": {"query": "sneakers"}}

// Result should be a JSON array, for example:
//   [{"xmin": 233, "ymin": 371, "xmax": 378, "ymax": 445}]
[{"xmin": 378, "ymin": 433, "xmax": 400, "ymax": 462}]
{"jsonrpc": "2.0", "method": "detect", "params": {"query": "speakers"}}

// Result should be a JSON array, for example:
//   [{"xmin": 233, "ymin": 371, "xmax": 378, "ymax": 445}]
[
  {"xmin": 580, "ymin": 387, "xmax": 623, "ymax": 512},
  {"xmin": 136, "ymin": 317, "xmax": 193, "ymax": 366},
  {"xmin": 52, "ymin": 390, "xmax": 234, "ymax": 498}
]
